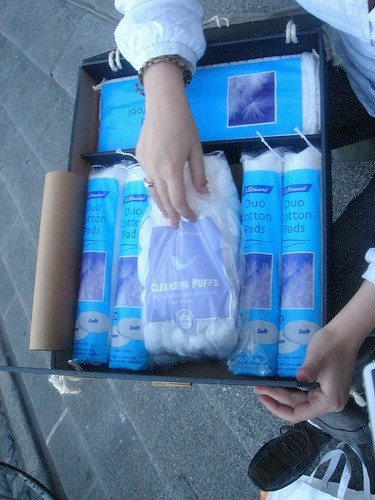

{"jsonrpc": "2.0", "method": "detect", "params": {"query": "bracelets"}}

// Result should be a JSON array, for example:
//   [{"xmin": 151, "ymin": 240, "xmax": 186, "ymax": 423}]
[{"xmin": 135, "ymin": 54, "xmax": 193, "ymax": 97}]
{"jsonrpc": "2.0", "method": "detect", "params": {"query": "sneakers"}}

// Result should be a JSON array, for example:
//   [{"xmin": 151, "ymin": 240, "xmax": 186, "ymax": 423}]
[{"xmin": 247, "ymin": 420, "xmax": 333, "ymax": 491}]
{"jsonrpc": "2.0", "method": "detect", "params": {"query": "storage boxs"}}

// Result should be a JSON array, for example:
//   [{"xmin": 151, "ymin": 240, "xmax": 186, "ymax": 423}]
[{"xmin": 29, "ymin": 14, "xmax": 331, "ymax": 388}]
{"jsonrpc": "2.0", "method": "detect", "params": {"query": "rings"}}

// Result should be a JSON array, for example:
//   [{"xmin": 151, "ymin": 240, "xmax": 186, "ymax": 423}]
[{"xmin": 143, "ymin": 178, "xmax": 154, "ymax": 188}]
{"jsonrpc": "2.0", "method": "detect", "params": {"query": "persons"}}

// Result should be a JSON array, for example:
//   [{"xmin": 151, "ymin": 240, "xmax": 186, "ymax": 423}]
[{"xmin": 113, "ymin": 0, "xmax": 375, "ymax": 492}]
